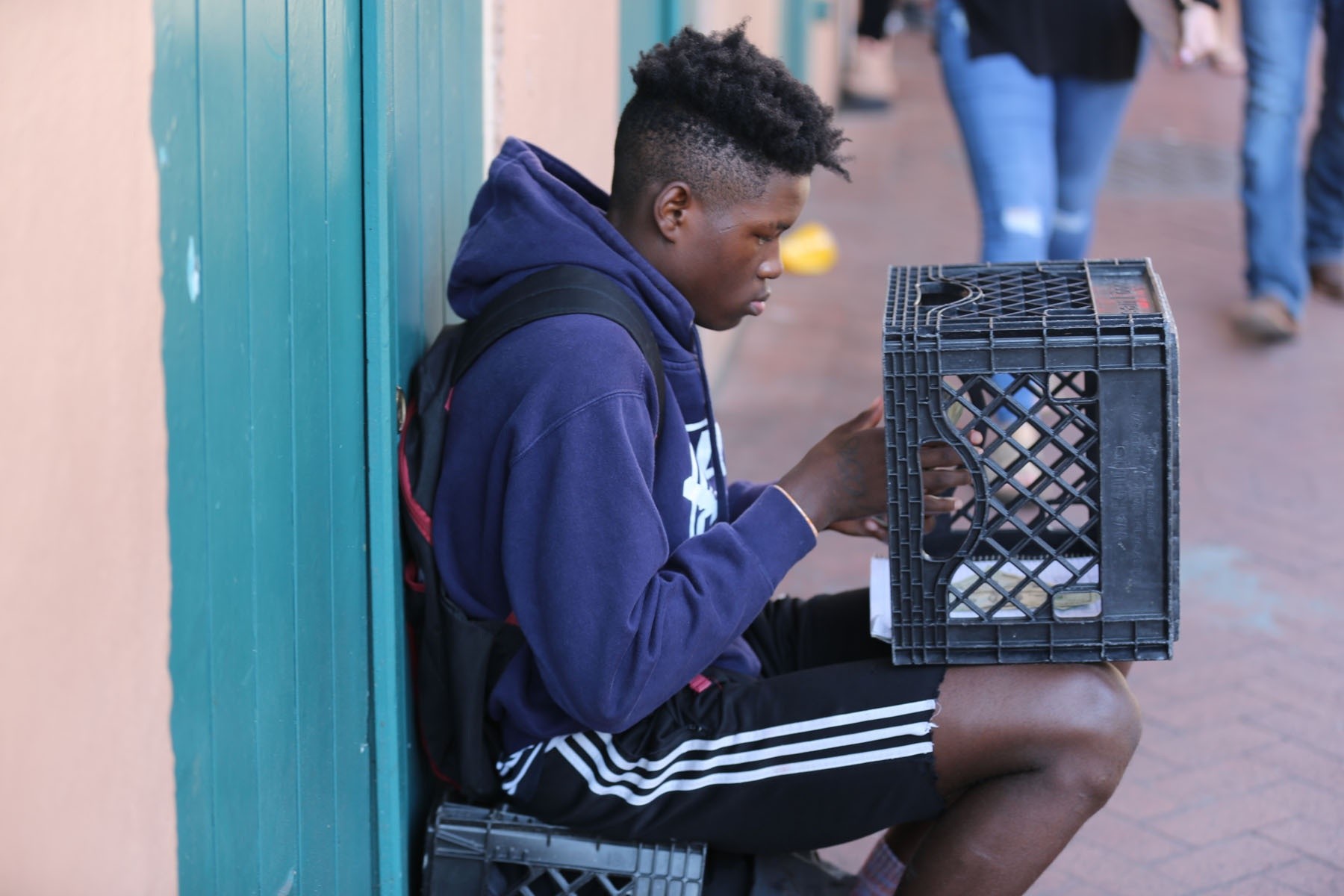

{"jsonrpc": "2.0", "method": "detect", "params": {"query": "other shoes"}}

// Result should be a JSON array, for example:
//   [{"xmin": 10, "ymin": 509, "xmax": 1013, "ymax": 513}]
[
  {"xmin": 1232, "ymin": 298, "xmax": 1297, "ymax": 338},
  {"xmin": 1312, "ymin": 262, "xmax": 1344, "ymax": 298}
]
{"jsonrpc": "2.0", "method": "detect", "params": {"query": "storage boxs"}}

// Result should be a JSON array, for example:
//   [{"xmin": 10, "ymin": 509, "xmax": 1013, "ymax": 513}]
[
  {"xmin": 420, "ymin": 797, "xmax": 709, "ymax": 895},
  {"xmin": 883, "ymin": 258, "xmax": 1182, "ymax": 667}
]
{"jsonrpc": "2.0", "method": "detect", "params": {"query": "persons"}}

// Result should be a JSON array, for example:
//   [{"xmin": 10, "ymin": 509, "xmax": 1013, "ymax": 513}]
[
  {"xmin": 398, "ymin": 16, "xmax": 1140, "ymax": 895},
  {"xmin": 844, "ymin": 0, "xmax": 1344, "ymax": 503}
]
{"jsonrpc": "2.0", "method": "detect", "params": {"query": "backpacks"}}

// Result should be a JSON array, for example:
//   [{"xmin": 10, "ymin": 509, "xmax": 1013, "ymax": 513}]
[{"xmin": 398, "ymin": 265, "xmax": 666, "ymax": 798}]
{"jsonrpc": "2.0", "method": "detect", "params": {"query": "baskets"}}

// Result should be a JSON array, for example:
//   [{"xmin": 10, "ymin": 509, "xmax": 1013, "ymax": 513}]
[
  {"xmin": 422, "ymin": 802, "xmax": 707, "ymax": 896},
  {"xmin": 883, "ymin": 258, "xmax": 1180, "ymax": 662}
]
